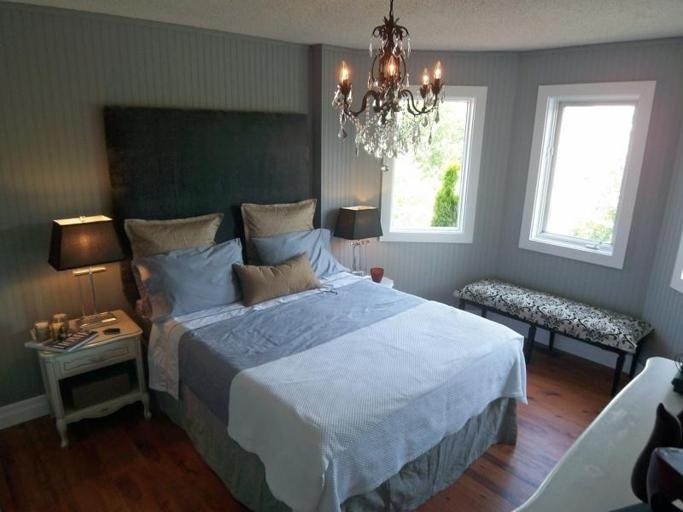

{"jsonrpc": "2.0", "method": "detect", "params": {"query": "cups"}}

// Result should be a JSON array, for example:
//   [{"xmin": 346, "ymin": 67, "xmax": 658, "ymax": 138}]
[
  {"xmin": 34, "ymin": 312, "xmax": 66, "ymax": 343},
  {"xmin": 369, "ymin": 267, "xmax": 384, "ymax": 283},
  {"xmin": 68, "ymin": 319, "xmax": 80, "ymax": 333}
]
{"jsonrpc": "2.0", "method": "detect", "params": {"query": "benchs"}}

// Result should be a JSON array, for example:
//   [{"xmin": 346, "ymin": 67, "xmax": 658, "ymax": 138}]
[{"xmin": 449, "ymin": 276, "xmax": 657, "ymax": 400}]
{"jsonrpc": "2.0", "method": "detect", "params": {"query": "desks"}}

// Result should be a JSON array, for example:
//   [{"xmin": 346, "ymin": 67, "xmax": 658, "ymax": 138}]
[{"xmin": 502, "ymin": 351, "xmax": 683, "ymax": 512}]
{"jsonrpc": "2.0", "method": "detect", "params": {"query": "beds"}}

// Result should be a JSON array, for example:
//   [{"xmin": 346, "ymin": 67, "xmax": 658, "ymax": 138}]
[{"xmin": 103, "ymin": 105, "xmax": 531, "ymax": 512}]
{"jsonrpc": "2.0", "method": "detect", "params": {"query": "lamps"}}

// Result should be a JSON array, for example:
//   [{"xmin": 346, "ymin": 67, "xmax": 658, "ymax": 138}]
[
  {"xmin": 47, "ymin": 213, "xmax": 127, "ymax": 329},
  {"xmin": 327, "ymin": 0, "xmax": 447, "ymax": 174},
  {"xmin": 333, "ymin": 204, "xmax": 384, "ymax": 279}
]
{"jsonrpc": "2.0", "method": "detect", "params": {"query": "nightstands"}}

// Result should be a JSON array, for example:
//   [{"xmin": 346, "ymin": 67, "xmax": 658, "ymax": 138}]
[
  {"xmin": 28, "ymin": 308, "xmax": 156, "ymax": 450},
  {"xmin": 351, "ymin": 271, "xmax": 395, "ymax": 291}
]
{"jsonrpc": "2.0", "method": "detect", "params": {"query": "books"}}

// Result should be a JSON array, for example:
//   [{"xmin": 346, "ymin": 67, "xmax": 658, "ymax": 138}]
[{"xmin": 24, "ymin": 327, "xmax": 96, "ymax": 353}]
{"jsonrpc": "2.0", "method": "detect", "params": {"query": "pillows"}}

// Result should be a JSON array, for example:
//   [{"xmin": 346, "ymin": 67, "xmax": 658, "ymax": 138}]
[
  {"xmin": 128, "ymin": 235, "xmax": 248, "ymax": 324},
  {"xmin": 123, "ymin": 211, "xmax": 229, "ymax": 262},
  {"xmin": 243, "ymin": 197, "xmax": 319, "ymax": 265},
  {"xmin": 247, "ymin": 222, "xmax": 354, "ymax": 281},
  {"xmin": 230, "ymin": 250, "xmax": 322, "ymax": 306}
]
